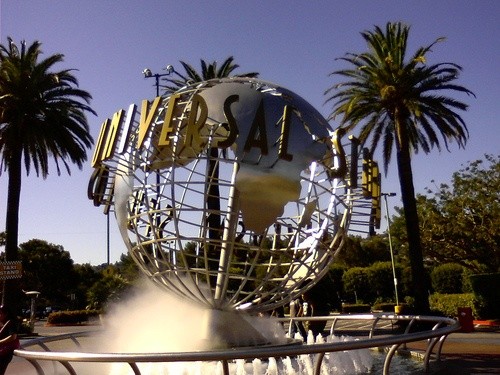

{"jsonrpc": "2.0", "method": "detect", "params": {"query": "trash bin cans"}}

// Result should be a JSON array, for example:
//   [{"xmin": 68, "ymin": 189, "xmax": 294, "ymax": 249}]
[{"xmin": 457, "ymin": 307, "xmax": 473, "ymax": 333}]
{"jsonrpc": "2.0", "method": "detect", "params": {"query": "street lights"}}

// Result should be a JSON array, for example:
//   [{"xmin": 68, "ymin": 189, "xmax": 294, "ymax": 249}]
[
  {"xmin": 144, "ymin": 65, "xmax": 174, "ymax": 208},
  {"xmin": 381, "ymin": 192, "xmax": 407, "ymax": 314}
]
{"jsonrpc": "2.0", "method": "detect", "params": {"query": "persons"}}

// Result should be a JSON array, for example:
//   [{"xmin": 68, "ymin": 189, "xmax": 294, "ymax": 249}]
[
  {"xmin": 288, "ymin": 294, "xmax": 317, "ymax": 343},
  {"xmin": 271, "ymin": 300, "xmax": 286, "ymax": 330},
  {"xmin": 0, "ymin": 304, "xmax": 20, "ymax": 375}
]
